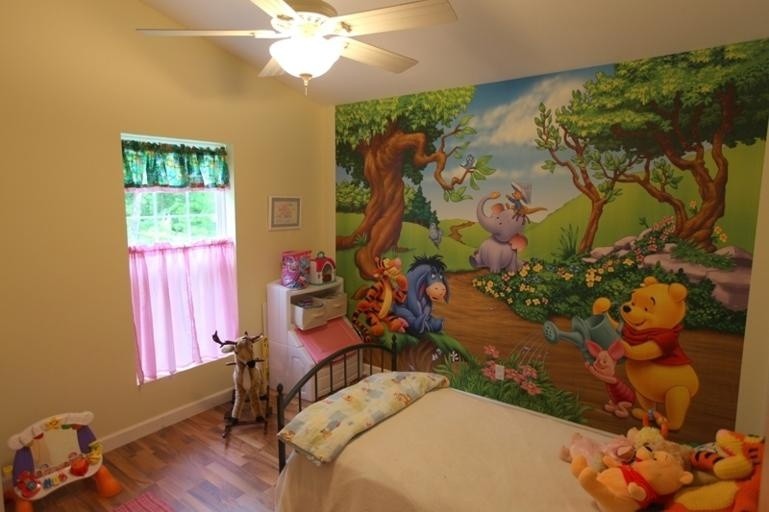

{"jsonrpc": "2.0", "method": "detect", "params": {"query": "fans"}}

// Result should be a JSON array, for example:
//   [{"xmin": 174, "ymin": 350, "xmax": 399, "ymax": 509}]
[{"xmin": 135, "ymin": 1, "xmax": 458, "ymax": 78}]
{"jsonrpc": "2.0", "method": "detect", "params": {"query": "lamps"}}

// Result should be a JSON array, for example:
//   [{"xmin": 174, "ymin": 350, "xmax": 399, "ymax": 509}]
[{"xmin": 269, "ymin": 19, "xmax": 347, "ymax": 93}]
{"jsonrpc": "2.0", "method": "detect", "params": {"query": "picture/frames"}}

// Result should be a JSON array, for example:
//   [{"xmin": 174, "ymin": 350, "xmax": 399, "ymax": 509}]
[{"xmin": 268, "ymin": 194, "xmax": 303, "ymax": 231}]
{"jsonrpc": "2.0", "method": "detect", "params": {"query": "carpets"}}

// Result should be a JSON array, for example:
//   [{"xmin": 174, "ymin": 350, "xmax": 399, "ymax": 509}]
[{"xmin": 114, "ymin": 491, "xmax": 177, "ymax": 512}]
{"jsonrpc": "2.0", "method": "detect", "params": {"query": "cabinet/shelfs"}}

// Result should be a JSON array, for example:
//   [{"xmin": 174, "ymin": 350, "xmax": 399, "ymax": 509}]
[{"xmin": 265, "ymin": 275, "xmax": 363, "ymax": 402}]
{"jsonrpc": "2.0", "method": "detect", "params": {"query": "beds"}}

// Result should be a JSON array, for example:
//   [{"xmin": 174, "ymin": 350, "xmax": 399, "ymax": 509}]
[{"xmin": 274, "ymin": 333, "xmax": 769, "ymax": 512}]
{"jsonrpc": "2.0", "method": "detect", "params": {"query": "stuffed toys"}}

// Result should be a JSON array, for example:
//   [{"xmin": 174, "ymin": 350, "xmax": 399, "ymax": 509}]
[{"xmin": 555, "ymin": 422, "xmax": 769, "ymax": 511}]
